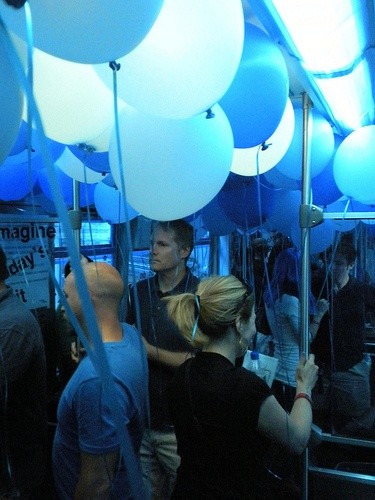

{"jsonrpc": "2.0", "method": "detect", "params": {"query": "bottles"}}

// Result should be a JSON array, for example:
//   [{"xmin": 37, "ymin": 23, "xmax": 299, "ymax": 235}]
[{"xmin": 246, "ymin": 351, "xmax": 266, "ymax": 382}]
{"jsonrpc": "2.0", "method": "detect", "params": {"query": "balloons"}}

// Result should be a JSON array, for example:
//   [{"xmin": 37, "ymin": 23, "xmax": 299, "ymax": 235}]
[{"xmin": 0, "ymin": 0, "xmax": 375, "ymax": 254}]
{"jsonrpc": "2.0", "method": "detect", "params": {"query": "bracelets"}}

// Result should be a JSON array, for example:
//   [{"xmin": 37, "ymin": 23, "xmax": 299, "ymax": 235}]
[{"xmin": 294, "ymin": 393, "xmax": 313, "ymax": 406}]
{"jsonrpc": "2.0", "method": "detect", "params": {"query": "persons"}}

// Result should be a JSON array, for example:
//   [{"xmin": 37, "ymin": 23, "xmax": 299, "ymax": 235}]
[{"xmin": 0, "ymin": 218, "xmax": 375, "ymax": 500}]
